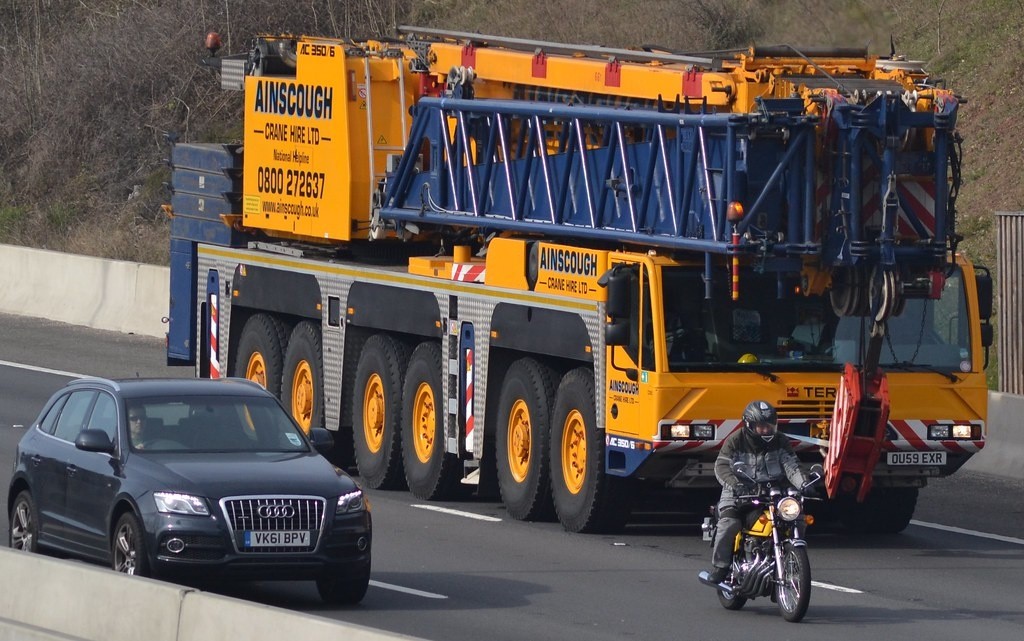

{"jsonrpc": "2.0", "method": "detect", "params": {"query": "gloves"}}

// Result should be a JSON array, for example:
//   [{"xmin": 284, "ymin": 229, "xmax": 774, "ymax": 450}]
[
  {"xmin": 733, "ymin": 483, "xmax": 750, "ymax": 496},
  {"xmin": 802, "ymin": 482, "xmax": 817, "ymax": 497}
]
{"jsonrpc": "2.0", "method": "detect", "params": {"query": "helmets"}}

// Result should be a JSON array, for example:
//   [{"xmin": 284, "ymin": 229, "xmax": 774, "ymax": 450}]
[{"xmin": 741, "ymin": 401, "xmax": 778, "ymax": 453}]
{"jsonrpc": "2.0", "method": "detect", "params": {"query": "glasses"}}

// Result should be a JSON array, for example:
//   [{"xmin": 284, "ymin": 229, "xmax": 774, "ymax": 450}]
[{"xmin": 128, "ymin": 415, "xmax": 147, "ymax": 422}]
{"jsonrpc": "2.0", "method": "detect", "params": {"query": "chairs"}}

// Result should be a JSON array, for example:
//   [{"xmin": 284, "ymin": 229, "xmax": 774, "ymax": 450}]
[{"xmin": 145, "ymin": 415, "xmax": 193, "ymax": 446}]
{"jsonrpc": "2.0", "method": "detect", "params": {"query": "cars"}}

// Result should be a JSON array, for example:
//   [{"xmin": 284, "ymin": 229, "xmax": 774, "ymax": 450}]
[{"xmin": 7, "ymin": 373, "xmax": 376, "ymax": 607}]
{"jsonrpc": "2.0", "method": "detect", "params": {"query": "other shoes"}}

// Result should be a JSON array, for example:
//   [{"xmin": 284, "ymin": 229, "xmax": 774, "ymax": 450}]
[{"xmin": 707, "ymin": 565, "xmax": 729, "ymax": 584}]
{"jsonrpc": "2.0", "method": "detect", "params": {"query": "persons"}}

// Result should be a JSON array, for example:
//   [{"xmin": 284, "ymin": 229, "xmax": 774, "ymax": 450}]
[
  {"xmin": 118, "ymin": 402, "xmax": 149, "ymax": 451},
  {"xmin": 707, "ymin": 399, "xmax": 823, "ymax": 584}
]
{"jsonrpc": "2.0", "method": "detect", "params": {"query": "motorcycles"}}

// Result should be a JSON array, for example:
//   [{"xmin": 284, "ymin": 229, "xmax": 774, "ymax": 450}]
[{"xmin": 694, "ymin": 460, "xmax": 831, "ymax": 625}]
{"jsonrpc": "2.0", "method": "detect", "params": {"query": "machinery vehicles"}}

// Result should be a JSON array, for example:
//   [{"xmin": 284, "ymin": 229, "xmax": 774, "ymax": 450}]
[{"xmin": 159, "ymin": 27, "xmax": 998, "ymax": 533}]
